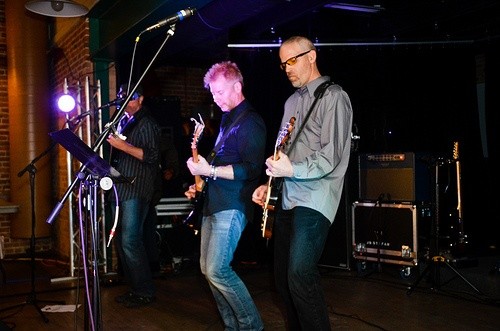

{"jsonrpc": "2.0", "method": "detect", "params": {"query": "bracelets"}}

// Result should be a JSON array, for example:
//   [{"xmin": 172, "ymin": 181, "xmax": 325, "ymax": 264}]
[{"xmin": 210, "ymin": 164, "xmax": 216, "ymax": 181}]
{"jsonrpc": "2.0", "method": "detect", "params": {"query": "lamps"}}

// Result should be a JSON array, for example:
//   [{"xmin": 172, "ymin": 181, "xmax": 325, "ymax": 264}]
[{"xmin": 24, "ymin": 0, "xmax": 89, "ymax": 18}]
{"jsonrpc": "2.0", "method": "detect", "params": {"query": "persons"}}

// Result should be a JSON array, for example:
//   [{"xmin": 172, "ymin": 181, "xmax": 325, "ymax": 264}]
[
  {"xmin": 186, "ymin": 62, "xmax": 268, "ymax": 331},
  {"xmin": 252, "ymin": 35, "xmax": 353, "ymax": 331},
  {"xmin": 105, "ymin": 83, "xmax": 179, "ymax": 307}
]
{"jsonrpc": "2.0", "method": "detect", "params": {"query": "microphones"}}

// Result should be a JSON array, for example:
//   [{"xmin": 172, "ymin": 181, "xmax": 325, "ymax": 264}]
[
  {"xmin": 103, "ymin": 93, "xmax": 140, "ymax": 109},
  {"xmin": 70, "ymin": 110, "xmax": 93, "ymax": 122},
  {"xmin": 146, "ymin": 6, "xmax": 196, "ymax": 31},
  {"xmin": 375, "ymin": 194, "xmax": 384, "ymax": 205}
]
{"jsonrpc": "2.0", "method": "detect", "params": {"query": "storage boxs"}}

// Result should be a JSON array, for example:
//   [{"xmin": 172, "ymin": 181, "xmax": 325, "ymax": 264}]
[{"xmin": 350, "ymin": 201, "xmax": 420, "ymax": 266}]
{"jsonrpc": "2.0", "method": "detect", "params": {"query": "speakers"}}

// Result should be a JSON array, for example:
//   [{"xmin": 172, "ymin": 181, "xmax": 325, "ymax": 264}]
[
  {"xmin": 319, "ymin": 175, "xmax": 352, "ymax": 272},
  {"xmin": 359, "ymin": 152, "xmax": 415, "ymax": 202}
]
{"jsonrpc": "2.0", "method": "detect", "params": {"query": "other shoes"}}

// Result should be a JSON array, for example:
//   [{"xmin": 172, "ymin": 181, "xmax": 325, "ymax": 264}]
[
  {"xmin": 115, "ymin": 292, "xmax": 134, "ymax": 304},
  {"xmin": 125, "ymin": 295, "xmax": 158, "ymax": 307}
]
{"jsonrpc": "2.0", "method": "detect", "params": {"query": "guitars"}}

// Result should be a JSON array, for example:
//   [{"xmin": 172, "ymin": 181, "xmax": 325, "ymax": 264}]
[
  {"xmin": 261, "ymin": 116, "xmax": 297, "ymax": 238},
  {"xmin": 451, "ymin": 139, "xmax": 465, "ymax": 235},
  {"xmin": 183, "ymin": 113, "xmax": 208, "ymax": 226}
]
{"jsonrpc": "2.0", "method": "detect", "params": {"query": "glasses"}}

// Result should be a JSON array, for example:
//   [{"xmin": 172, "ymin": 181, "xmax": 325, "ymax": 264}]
[{"xmin": 279, "ymin": 49, "xmax": 316, "ymax": 70}]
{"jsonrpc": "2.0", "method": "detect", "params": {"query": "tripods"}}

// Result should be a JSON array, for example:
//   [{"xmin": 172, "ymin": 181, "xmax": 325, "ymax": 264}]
[
  {"xmin": 407, "ymin": 161, "xmax": 482, "ymax": 299},
  {"xmin": 0, "ymin": 107, "xmax": 103, "ymax": 323}
]
